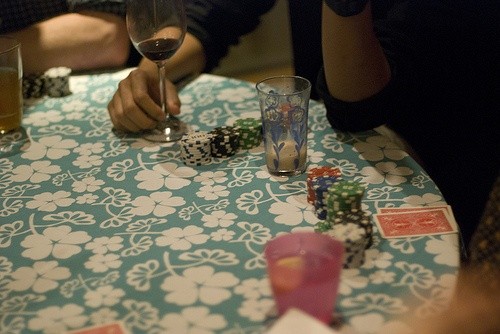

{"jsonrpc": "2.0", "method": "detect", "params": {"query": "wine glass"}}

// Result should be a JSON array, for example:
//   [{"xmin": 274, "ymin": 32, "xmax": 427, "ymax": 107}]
[{"xmin": 125, "ymin": 0, "xmax": 187, "ymax": 142}]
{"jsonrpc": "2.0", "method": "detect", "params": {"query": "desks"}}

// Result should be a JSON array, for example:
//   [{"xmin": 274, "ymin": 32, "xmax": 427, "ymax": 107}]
[{"xmin": 0, "ymin": 68, "xmax": 468, "ymax": 334}]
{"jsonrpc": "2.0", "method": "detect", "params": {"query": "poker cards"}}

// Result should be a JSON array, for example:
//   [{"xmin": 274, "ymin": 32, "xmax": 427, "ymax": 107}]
[
  {"xmin": 75, "ymin": 320, "xmax": 129, "ymax": 334},
  {"xmin": 373, "ymin": 204, "xmax": 458, "ymax": 239}
]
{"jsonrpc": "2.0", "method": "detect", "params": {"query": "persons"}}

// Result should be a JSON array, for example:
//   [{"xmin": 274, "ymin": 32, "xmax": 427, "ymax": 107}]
[
  {"xmin": 107, "ymin": 0, "xmax": 500, "ymax": 264},
  {"xmin": 0, "ymin": 0, "xmax": 143, "ymax": 77}
]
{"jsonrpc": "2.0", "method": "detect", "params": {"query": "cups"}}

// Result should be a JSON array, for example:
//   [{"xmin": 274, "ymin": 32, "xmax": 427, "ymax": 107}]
[
  {"xmin": 256, "ymin": 76, "xmax": 311, "ymax": 176},
  {"xmin": 0, "ymin": 38, "xmax": 23, "ymax": 135},
  {"xmin": 266, "ymin": 232, "xmax": 344, "ymax": 326}
]
